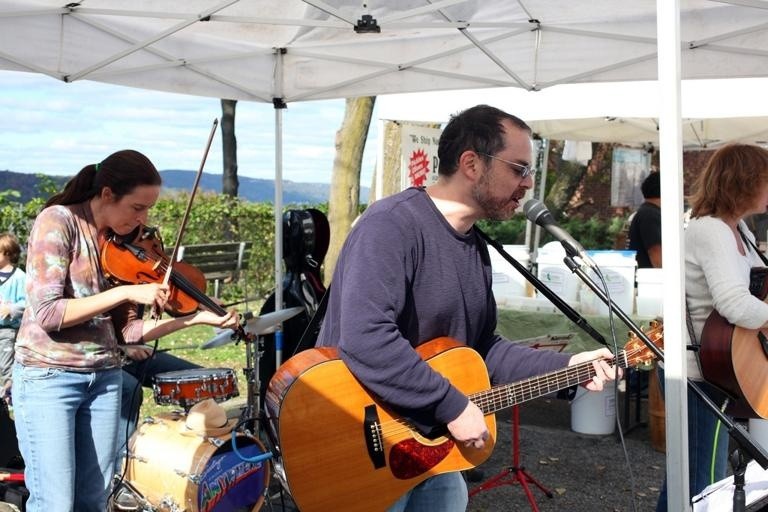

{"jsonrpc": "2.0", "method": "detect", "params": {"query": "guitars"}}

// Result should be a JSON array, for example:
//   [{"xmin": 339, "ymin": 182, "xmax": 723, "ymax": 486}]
[
  {"xmin": 700, "ymin": 267, "xmax": 767, "ymax": 420},
  {"xmin": 264, "ymin": 318, "xmax": 663, "ymax": 511}
]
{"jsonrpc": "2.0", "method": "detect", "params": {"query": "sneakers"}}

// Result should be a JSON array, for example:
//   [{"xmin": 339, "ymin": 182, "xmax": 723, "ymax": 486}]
[{"xmin": 113, "ymin": 483, "xmax": 141, "ymax": 510}]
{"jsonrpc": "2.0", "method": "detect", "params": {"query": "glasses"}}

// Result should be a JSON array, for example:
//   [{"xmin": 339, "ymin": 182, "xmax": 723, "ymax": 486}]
[{"xmin": 483, "ymin": 153, "xmax": 536, "ymax": 178}]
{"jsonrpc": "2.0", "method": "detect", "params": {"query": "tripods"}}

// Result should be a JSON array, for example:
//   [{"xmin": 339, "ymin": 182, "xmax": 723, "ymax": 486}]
[{"xmin": 467, "ymin": 404, "xmax": 553, "ymax": 512}]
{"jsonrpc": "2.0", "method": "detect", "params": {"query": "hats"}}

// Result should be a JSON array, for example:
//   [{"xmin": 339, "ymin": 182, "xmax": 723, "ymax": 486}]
[{"xmin": 179, "ymin": 398, "xmax": 238, "ymax": 437}]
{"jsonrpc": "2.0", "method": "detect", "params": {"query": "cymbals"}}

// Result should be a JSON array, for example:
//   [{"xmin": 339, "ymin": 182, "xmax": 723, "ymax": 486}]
[{"xmin": 200, "ymin": 307, "xmax": 305, "ymax": 348}]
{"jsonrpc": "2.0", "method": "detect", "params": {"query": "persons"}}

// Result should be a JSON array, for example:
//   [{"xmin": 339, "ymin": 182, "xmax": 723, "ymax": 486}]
[
  {"xmin": 12, "ymin": 149, "xmax": 240, "ymax": 512},
  {"xmin": 617, "ymin": 211, "xmax": 636, "ymax": 249},
  {"xmin": 0, "ymin": 231, "xmax": 27, "ymax": 400},
  {"xmin": 316, "ymin": 105, "xmax": 625, "ymax": 512},
  {"xmin": 112, "ymin": 345, "xmax": 206, "ymax": 511},
  {"xmin": 654, "ymin": 145, "xmax": 768, "ymax": 512},
  {"xmin": 628, "ymin": 172, "xmax": 662, "ymax": 267}
]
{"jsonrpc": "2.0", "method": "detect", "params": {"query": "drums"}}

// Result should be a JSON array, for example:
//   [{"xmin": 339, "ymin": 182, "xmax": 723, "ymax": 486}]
[
  {"xmin": 154, "ymin": 369, "xmax": 239, "ymax": 407},
  {"xmin": 122, "ymin": 413, "xmax": 270, "ymax": 512}
]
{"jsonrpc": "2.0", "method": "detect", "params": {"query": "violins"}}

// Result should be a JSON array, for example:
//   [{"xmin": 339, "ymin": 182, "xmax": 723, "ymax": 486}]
[{"xmin": 101, "ymin": 222, "xmax": 257, "ymax": 346}]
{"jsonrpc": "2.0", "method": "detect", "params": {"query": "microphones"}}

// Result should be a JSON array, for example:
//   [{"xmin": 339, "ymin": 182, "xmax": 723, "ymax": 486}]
[{"xmin": 523, "ymin": 200, "xmax": 596, "ymax": 267}]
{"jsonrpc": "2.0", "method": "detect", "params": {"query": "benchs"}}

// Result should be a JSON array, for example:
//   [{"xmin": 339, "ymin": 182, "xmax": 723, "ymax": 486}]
[{"xmin": 164, "ymin": 241, "xmax": 253, "ymax": 298}]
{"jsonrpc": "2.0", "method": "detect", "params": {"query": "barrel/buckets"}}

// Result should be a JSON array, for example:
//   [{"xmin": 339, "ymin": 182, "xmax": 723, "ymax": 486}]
[
  {"xmin": 570, "ymin": 381, "xmax": 616, "ymax": 435},
  {"xmin": 648, "ymin": 368, "xmax": 667, "ymax": 454}
]
